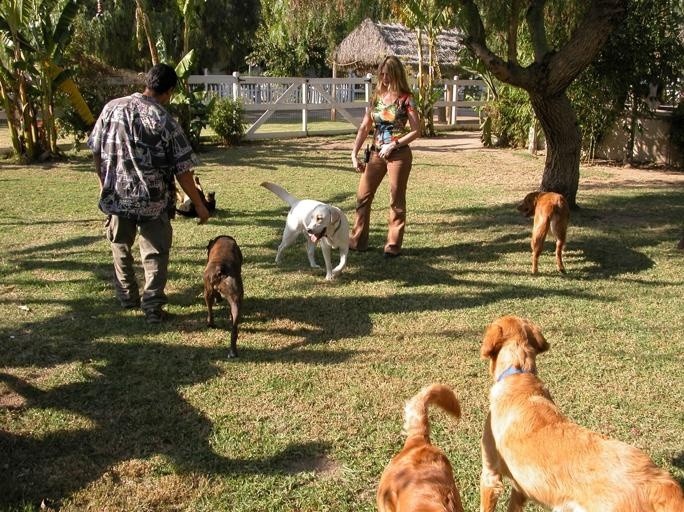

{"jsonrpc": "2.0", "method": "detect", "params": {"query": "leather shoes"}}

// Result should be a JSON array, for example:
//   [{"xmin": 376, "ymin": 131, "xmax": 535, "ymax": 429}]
[{"xmin": 383, "ymin": 253, "xmax": 393, "ymax": 258}]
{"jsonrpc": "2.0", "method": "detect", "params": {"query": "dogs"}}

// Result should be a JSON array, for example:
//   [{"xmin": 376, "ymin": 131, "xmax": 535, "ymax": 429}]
[
  {"xmin": 375, "ymin": 382, "xmax": 465, "ymax": 512},
  {"xmin": 259, "ymin": 181, "xmax": 350, "ymax": 283},
  {"xmin": 203, "ymin": 234, "xmax": 244, "ymax": 358},
  {"xmin": 175, "ymin": 170, "xmax": 216, "ymax": 218},
  {"xmin": 516, "ymin": 191, "xmax": 570, "ymax": 274},
  {"xmin": 479, "ymin": 315, "xmax": 684, "ymax": 512}
]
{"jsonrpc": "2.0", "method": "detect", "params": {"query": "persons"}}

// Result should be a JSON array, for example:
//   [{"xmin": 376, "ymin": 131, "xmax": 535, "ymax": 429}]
[
  {"xmin": 90, "ymin": 63, "xmax": 209, "ymax": 326},
  {"xmin": 349, "ymin": 55, "xmax": 421, "ymax": 258}
]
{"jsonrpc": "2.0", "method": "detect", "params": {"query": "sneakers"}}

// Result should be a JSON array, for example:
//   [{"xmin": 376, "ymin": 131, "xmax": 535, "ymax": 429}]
[{"xmin": 146, "ymin": 308, "xmax": 165, "ymax": 323}]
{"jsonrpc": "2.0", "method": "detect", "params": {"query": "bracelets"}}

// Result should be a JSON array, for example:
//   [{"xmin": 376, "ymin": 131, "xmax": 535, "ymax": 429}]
[{"xmin": 394, "ymin": 139, "xmax": 401, "ymax": 149}]
